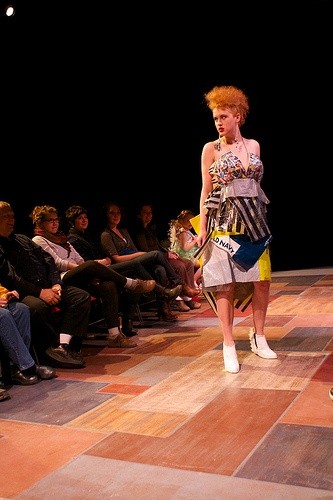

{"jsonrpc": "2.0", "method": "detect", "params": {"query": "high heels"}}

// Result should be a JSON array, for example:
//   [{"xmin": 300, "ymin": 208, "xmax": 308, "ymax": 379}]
[
  {"xmin": 174, "ymin": 284, "xmax": 203, "ymax": 298},
  {"xmin": 170, "ymin": 299, "xmax": 202, "ymax": 311},
  {"xmin": 157, "ymin": 307, "xmax": 178, "ymax": 322}
]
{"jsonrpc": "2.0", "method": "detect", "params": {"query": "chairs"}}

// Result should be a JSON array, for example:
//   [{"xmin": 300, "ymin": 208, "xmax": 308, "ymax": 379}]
[{"xmin": 30, "ymin": 292, "xmax": 157, "ymax": 365}]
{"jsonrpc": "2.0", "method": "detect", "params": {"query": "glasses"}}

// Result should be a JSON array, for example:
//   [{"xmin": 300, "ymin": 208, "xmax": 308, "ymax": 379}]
[{"xmin": 43, "ymin": 217, "xmax": 60, "ymax": 223}]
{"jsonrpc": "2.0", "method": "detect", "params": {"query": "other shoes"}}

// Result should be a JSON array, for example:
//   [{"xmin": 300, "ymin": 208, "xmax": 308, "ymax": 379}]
[
  {"xmin": 121, "ymin": 327, "xmax": 139, "ymax": 336},
  {"xmin": 0, "ymin": 389, "xmax": 10, "ymax": 401}
]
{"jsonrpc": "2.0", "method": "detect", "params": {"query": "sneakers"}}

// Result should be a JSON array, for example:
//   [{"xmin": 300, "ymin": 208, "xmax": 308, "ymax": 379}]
[
  {"xmin": 248, "ymin": 330, "xmax": 278, "ymax": 360},
  {"xmin": 223, "ymin": 342, "xmax": 240, "ymax": 373}
]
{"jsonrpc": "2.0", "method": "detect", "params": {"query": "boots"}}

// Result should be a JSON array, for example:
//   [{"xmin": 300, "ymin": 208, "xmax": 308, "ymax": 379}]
[{"xmin": 152, "ymin": 284, "xmax": 182, "ymax": 305}]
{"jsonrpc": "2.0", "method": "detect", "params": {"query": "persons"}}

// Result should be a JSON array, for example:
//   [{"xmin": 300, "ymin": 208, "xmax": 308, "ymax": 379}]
[
  {"xmin": 197, "ymin": 86, "xmax": 278, "ymax": 373},
  {"xmin": 0, "ymin": 202, "xmax": 204, "ymax": 401}
]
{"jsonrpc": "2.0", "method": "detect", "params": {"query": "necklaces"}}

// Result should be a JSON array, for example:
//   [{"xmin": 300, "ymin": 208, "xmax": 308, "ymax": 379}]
[{"xmin": 224, "ymin": 133, "xmax": 240, "ymax": 149}]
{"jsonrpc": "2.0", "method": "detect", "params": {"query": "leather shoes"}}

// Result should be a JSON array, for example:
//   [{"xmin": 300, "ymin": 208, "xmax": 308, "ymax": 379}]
[
  {"xmin": 126, "ymin": 279, "xmax": 156, "ymax": 293},
  {"xmin": 27, "ymin": 364, "xmax": 57, "ymax": 380},
  {"xmin": 5, "ymin": 370, "xmax": 39, "ymax": 385},
  {"xmin": 48, "ymin": 345, "xmax": 87, "ymax": 368},
  {"xmin": 107, "ymin": 333, "xmax": 138, "ymax": 348}
]
